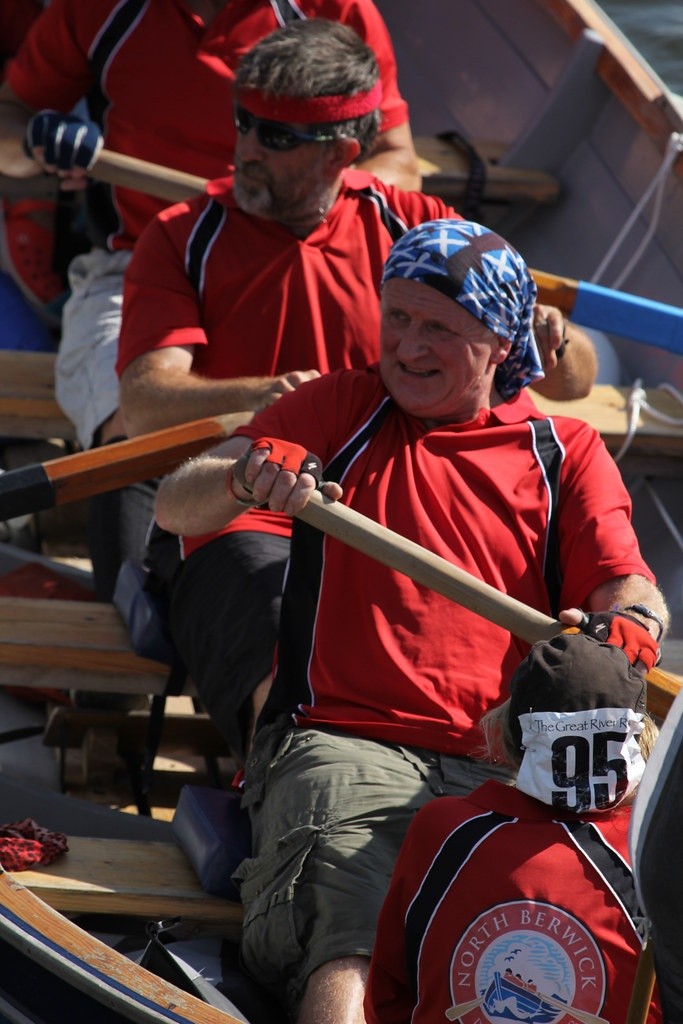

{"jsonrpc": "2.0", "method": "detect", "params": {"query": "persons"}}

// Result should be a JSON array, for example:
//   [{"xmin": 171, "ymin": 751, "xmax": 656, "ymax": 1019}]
[
  {"xmin": 361, "ymin": 634, "xmax": 672, "ymax": 1023},
  {"xmin": 0, "ymin": 0, "xmax": 423, "ymax": 452},
  {"xmin": 151, "ymin": 214, "xmax": 670, "ymax": 1024},
  {"xmin": 115, "ymin": 18, "xmax": 602, "ymax": 789}
]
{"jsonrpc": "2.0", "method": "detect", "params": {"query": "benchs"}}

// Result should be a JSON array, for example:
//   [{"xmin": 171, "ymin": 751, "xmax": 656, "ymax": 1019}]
[
  {"xmin": 0, "ymin": 598, "xmax": 197, "ymax": 791},
  {"xmin": 10, "ymin": 835, "xmax": 246, "ymax": 957},
  {"xmin": 0, "ymin": 349, "xmax": 683, "ymax": 478},
  {"xmin": 410, "ymin": 132, "xmax": 563, "ymax": 233}
]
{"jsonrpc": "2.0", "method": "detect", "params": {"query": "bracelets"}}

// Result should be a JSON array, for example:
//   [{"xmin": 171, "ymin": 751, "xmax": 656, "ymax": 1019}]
[
  {"xmin": 625, "ymin": 602, "xmax": 665, "ymax": 643},
  {"xmin": 556, "ymin": 321, "xmax": 568, "ymax": 359},
  {"xmin": 225, "ymin": 462, "xmax": 269, "ymax": 507}
]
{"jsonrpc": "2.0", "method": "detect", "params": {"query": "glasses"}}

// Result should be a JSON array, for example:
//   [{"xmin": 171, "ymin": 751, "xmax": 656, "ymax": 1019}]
[{"xmin": 233, "ymin": 101, "xmax": 347, "ymax": 152}]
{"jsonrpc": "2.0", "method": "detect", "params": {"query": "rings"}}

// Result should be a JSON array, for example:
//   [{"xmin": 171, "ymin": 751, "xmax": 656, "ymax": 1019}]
[{"xmin": 535, "ymin": 321, "xmax": 548, "ymax": 329}]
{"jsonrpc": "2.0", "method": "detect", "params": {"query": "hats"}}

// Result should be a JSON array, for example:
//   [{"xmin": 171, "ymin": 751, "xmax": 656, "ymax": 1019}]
[{"xmin": 508, "ymin": 634, "xmax": 649, "ymax": 757}]
